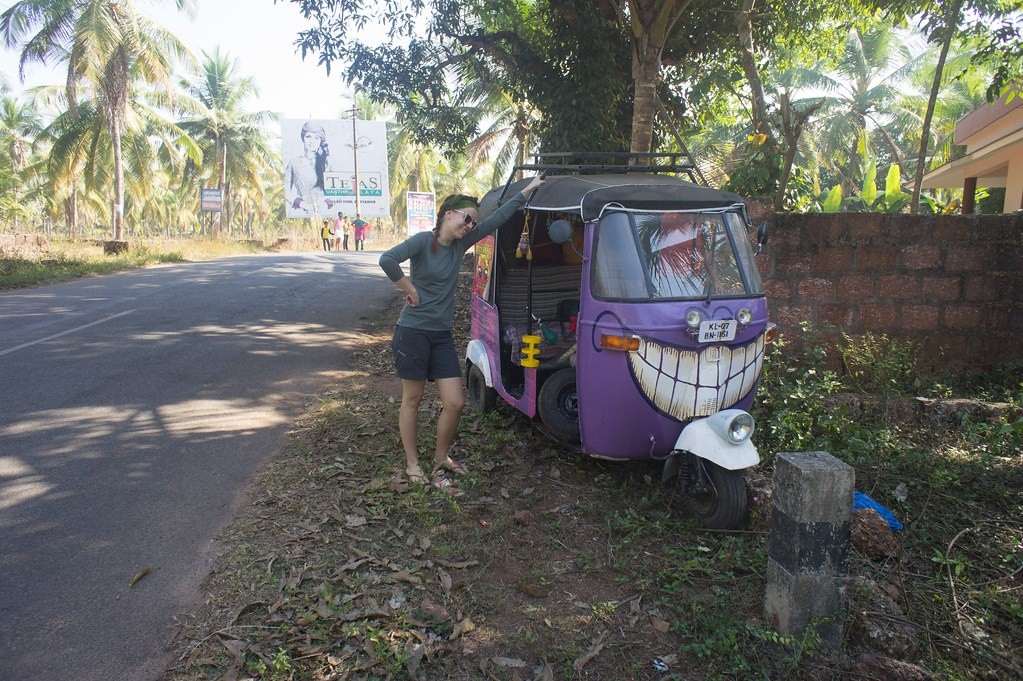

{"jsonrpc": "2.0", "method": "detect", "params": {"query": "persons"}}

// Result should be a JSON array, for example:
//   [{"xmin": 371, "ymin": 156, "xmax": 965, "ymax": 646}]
[
  {"xmin": 321, "ymin": 212, "xmax": 369, "ymax": 252},
  {"xmin": 378, "ymin": 174, "xmax": 547, "ymax": 488}
]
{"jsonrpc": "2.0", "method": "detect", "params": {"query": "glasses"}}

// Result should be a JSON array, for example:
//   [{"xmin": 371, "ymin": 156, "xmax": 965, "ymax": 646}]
[{"xmin": 452, "ymin": 208, "xmax": 477, "ymax": 230}]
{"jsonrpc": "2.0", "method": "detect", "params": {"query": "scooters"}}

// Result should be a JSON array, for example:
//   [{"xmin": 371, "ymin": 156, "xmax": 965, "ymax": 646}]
[{"xmin": 460, "ymin": 151, "xmax": 774, "ymax": 530}]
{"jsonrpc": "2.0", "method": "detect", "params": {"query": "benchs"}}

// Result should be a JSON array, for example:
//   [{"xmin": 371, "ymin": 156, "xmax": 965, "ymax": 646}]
[{"xmin": 498, "ymin": 264, "xmax": 644, "ymax": 367}]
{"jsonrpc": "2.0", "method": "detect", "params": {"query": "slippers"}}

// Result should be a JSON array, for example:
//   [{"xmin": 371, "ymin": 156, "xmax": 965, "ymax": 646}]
[
  {"xmin": 432, "ymin": 457, "xmax": 469, "ymax": 475},
  {"xmin": 406, "ymin": 467, "xmax": 430, "ymax": 485}
]
